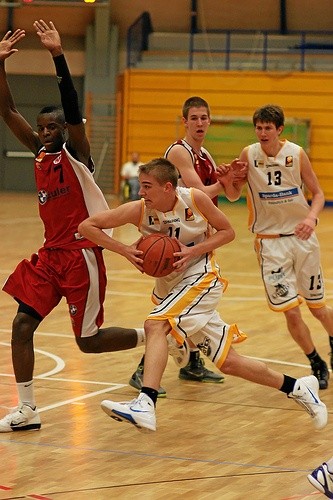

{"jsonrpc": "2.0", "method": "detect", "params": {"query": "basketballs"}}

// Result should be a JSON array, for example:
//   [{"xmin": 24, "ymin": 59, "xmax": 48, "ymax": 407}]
[{"xmin": 139, "ymin": 232, "xmax": 181, "ymax": 279}]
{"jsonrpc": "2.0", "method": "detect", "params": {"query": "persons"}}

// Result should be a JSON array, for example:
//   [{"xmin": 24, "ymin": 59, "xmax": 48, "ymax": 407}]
[
  {"xmin": 0, "ymin": 19, "xmax": 190, "ymax": 434},
  {"xmin": 76, "ymin": 96, "xmax": 333, "ymax": 434}
]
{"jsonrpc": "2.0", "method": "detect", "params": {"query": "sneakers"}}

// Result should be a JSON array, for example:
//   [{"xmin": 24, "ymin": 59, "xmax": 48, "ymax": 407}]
[
  {"xmin": 178, "ymin": 351, "xmax": 225, "ymax": 383},
  {"xmin": 0, "ymin": 404, "xmax": 41, "ymax": 432},
  {"xmin": 100, "ymin": 393, "xmax": 156, "ymax": 434},
  {"xmin": 307, "ymin": 463, "xmax": 333, "ymax": 500},
  {"xmin": 312, "ymin": 360, "xmax": 329, "ymax": 389},
  {"xmin": 129, "ymin": 355, "xmax": 168, "ymax": 398},
  {"xmin": 287, "ymin": 375, "xmax": 327, "ymax": 430}
]
